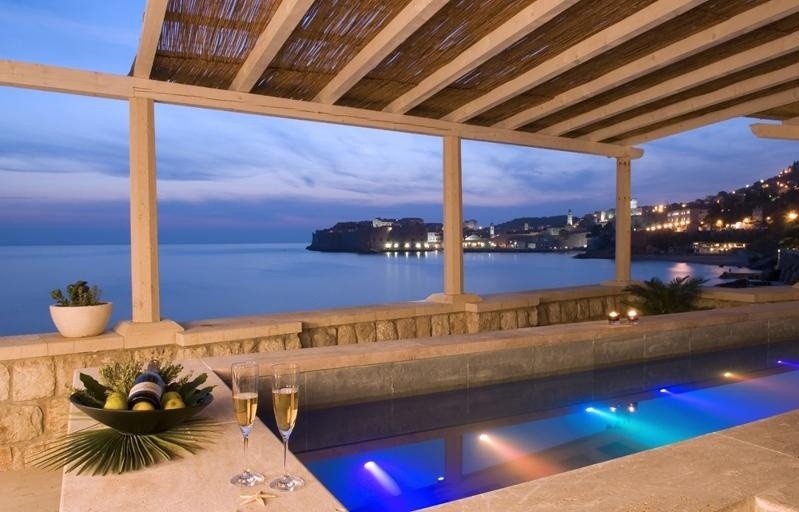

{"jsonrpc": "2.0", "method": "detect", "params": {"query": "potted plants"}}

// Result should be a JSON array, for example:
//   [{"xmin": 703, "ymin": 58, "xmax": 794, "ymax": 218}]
[{"xmin": 49, "ymin": 281, "xmax": 115, "ymax": 339}]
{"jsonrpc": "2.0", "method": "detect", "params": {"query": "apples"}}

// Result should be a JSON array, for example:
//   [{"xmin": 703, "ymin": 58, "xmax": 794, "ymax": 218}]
[{"xmin": 104, "ymin": 390, "xmax": 184, "ymax": 410}]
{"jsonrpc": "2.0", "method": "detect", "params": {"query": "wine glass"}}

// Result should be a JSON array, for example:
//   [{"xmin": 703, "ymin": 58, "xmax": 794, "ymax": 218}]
[
  {"xmin": 230, "ymin": 361, "xmax": 265, "ymax": 487},
  {"xmin": 267, "ymin": 362, "xmax": 304, "ymax": 492}
]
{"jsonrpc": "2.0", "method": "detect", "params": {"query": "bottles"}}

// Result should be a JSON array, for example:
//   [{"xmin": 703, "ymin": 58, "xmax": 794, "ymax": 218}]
[{"xmin": 126, "ymin": 358, "xmax": 166, "ymax": 410}]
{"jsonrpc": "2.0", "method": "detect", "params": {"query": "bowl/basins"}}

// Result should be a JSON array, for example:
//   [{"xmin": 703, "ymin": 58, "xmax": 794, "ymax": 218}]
[{"xmin": 70, "ymin": 384, "xmax": 214, "ymax": 436}]
